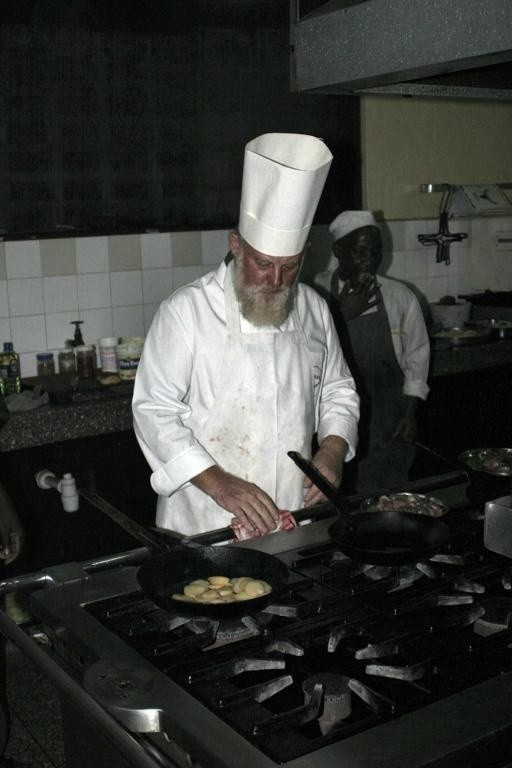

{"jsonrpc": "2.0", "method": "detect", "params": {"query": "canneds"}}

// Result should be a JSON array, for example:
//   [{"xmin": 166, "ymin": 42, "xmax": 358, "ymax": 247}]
[
  {"xmin": 36, "ymin": 353, "xmax": 55, "ymax": 376},
  {"xmin": 58, "ymin": 348, "xmax": 76, "ymax": 375},
  {"xmin": 76, "ymin": 345, "xmax": 97, "ymax": 370}
]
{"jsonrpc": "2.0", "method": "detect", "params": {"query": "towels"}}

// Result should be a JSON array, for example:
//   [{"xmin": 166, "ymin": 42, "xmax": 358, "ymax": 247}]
[{"xmin": 229, "ymin": 510, "xmax": 298, "ymax": 541}]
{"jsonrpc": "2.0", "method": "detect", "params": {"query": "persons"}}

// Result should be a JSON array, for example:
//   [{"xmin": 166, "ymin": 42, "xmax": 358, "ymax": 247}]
[
  {"xmin": 314, "ymin": 208, "xmax": 434, "ymax": 500},
  {"xmin": 129, "ymin": 224, "xmax": 363, "ymax": 540}
]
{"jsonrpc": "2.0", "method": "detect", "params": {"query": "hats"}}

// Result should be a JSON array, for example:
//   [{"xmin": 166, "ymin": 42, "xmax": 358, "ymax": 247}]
[
  {"xmin": 239, "ymin": 128, "xmax": 333, "ymax": 258},
  {"xmin": 327, "ymin": 208, "xmax": 381, "ymax": 240}
]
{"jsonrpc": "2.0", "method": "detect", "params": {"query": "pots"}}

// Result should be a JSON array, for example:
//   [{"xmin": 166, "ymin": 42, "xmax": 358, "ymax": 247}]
[
  {"xmin": 430, "ymin": 325, "xmax": 495, "ymax": 347},
  {"xmin": 136, "ymin": 527, "xmax": 290, "ymax": 619},
  {"xmin": 456, "ymin": 445, "xmax": 512, "ymax": 478},
  {"xmin": 375, "ymin": 492, "xmax": 451, "ymax": 521},
  {"xmin": 464, "ymin": 318, "xmax": 512, "ymax": 342},
  {"xmin": 287, "ymin": 451, "xmax": 453, "ymax": 566}
]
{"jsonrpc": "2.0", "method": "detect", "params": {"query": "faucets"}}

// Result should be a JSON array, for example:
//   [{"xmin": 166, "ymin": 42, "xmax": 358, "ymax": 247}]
[{"xmin": 64, "ymin": 319, "xmax": 85, "ymax": 348}]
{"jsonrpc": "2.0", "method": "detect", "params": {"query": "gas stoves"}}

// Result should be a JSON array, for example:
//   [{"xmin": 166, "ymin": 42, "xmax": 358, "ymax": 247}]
[{"xmin": 14, "ymin": 506, "xmax": 512, "ymax": 768}]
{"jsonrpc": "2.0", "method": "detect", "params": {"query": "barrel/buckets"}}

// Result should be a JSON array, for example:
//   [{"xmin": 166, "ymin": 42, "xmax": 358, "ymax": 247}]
[{"xmin": 428, "ymin": 301, "xmax": 473, "ymax": 329}]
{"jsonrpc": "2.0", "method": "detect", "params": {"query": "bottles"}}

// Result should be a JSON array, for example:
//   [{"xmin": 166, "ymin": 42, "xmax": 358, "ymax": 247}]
[{"xmin": 0, "ymin": 336, "xmax": 146, "ymax": 398}]
